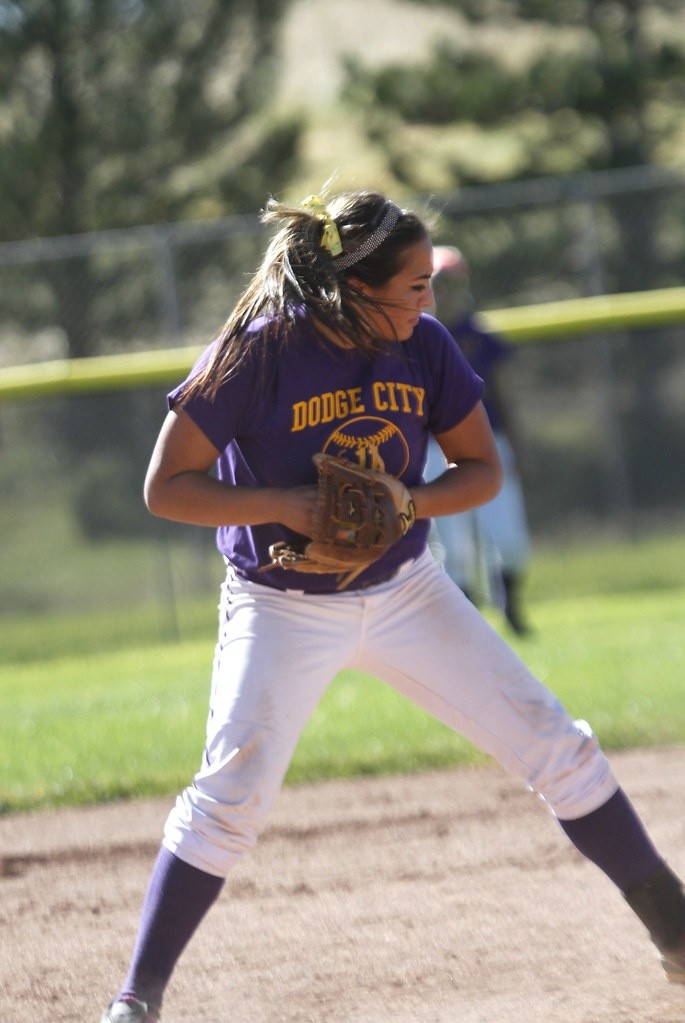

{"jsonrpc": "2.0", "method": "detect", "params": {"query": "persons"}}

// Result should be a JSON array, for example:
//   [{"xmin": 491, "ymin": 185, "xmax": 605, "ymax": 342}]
[
  {"xmin": 423, "ymin": 245, "xmax": 531, "ymax": 638},
  {"xmin": 101, "ymin": 192, "xmax": 685, "ymax": 1023}
]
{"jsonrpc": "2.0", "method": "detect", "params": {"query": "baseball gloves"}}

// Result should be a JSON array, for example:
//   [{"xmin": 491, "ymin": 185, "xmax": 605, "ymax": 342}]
[{"xmin": 269, "ymin": 453, "xmax": 415, "ymax": 574}]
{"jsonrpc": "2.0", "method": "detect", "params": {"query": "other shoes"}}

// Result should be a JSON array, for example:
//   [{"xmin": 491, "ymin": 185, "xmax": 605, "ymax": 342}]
[
  {"xmin": 101, "ymin": 997, "xmax": 160, "ymax": 1023},
  {"xmin": 498, "ymin": 607, "xmax": 528, "ymax": 638},
  {"xmin": 651, "ymin": 936, "xmax": 685, "ymax": 984}
]
